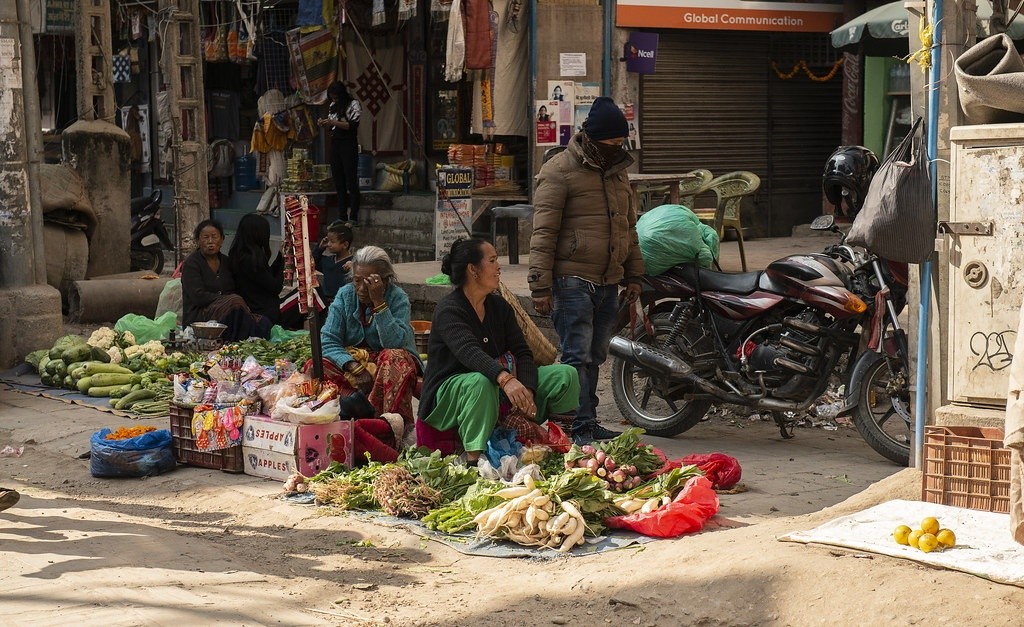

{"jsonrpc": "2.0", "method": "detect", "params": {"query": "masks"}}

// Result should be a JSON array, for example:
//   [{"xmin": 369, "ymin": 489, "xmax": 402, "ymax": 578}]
[{"xmin": 595, "ymin": 140, "xmax": 623, "ymax": 165}]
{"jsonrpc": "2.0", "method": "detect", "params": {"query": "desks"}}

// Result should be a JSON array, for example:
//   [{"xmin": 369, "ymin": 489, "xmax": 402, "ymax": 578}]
[{"xmin": 627, "ymin": 172, "xmax": 694, "ymax": 208}]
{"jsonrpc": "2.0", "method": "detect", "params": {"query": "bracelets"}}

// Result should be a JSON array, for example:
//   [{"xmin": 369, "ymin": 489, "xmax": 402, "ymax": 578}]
[
  {"xmin": 499, "ymin": 374, "xmax": 515, "ymax": 389},
  {"xmin": 373, "ymin": 302, "xmax": 387, "ymax": 313}
]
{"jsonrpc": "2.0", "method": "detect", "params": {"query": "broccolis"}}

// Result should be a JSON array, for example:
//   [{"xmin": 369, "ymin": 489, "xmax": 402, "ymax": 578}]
[{"xmin": 86, "ymin": 326, "xmax": 185, "ymax": 365}]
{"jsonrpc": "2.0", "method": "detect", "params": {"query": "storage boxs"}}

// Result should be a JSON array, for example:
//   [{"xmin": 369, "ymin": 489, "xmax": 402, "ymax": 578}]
[
  {"xmin": 242, "ymin": 415, "xmax": 353, "ymax": 482},
  {"xmin": 167, "ymin": 398, "xmax": 243, "ymax": 472},
  {"xmin": 922, "ymin": 423, "xmax": 1017, "ymax": 513}
]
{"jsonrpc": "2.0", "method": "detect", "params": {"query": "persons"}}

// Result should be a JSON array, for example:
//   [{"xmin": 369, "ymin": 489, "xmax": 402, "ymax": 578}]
[
  {"xmin": 182, "ymin": 213, "xmax": 304, "ymax": 343},
  {"xmin": 527, "ymin": 97, "xmax": 646, "ymax": 447},
  {"xmin": 311, "ymin": 219, "xmax": 356, "ymax": 304},
  {"xmin": 415, "ymin": 238, "xmax": 582, "ymax": 477},
  {"xmin": 300, "ymin": 246, "xmax": 425, "ymax": 438},
  {"xmin": 629, "ymin": 123, "xmax": 637, "ymax": 150},
  {"xmin": 537, "ymin": 105, "xmax": 554, "ymax": 121},
  {"xmin": 317, "ymin": 81, "xmax": 362, "ymax": 225},
  {"xmin": 551, "ymin": 86, "xmax": 568, "ymax": 101}
]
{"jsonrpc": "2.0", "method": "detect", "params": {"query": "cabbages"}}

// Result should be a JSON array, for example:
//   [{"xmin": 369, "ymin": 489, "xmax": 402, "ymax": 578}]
[{"xmin": 23, "ymin": 334, "xmax": 86, "ymax": 374}]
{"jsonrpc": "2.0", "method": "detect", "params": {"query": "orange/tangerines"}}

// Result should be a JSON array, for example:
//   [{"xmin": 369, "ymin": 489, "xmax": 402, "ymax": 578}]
[{"xmin": 894, "ymin": 518, "xmax": 956, "ymax": 551}]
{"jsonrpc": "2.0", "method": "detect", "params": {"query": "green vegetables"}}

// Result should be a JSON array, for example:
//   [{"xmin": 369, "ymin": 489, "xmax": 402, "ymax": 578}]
[
  {"xmin": 119, "ymin": 351, "xmax": 205, "ymax": 374},
  {"xmin": 537, "ymin": 451, "xmax": 567, "ymax": 478},
  {"xmin": 238, "ymin": 334, "xmax": 313, "ymax": 371},
  {"xmin": 308, "ymin": 444, "xmax": 521, "ymax": 535}
]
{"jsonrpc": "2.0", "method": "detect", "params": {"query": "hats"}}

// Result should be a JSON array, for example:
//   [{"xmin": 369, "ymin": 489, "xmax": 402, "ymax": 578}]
[{"xmin": 580, "ymin": 96, "xmax": 630, "ymax": 143}]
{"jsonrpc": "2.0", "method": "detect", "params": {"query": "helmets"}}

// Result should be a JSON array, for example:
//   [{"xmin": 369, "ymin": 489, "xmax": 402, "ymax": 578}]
[{"xmin": 823, "ymin": 144, "xmax": 880, "ymax": 210}]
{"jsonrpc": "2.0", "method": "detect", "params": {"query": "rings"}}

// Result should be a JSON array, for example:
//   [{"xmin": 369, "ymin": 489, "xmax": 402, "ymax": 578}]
[
  {"xmin": 532, "ymin": 413, "xmax": 536, "ymax": 416},
  {"xmin": 534, "ymin": 307, "xmax": 539, "ymax": 310},
  {"xmin": 375, "ymin": 279, "xmax": 378, "ymax": 282}
]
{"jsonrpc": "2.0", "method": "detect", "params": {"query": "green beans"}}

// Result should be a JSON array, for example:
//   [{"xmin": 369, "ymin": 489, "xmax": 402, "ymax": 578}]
[{"xmin": 132, "ymin": 387, "xmax": 175, "ymax": 417}]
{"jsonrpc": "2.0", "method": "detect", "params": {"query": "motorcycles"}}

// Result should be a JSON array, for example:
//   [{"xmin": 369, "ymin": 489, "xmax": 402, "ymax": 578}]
[{"xmin": 606, "ymin": 215, "xmax": 912, "ymax": 466}]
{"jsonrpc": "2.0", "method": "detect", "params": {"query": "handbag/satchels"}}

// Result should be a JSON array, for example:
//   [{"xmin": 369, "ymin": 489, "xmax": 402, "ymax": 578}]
[
  {"xmin": 89, "ymin": 428, "xmax": 177, "ymax": 478},
  {"xmin": 843, "ymin": 115, "xmax": 935, "ymax": 264}
]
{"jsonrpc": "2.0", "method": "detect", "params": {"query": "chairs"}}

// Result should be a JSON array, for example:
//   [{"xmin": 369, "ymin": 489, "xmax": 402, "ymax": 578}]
[
  {"xmin": 637, "ymin": 169, "xmax": 713, "ymax": 214},
  {"xmin": 685, "ymin": 170, "xmax": 761, "ymax": 273},
  {"xmin": 489, "ymin": 146, "xmax": 571, "ymax": 265}
]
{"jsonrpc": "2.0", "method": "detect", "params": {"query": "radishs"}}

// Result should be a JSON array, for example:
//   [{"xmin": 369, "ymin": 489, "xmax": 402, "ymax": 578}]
[
  {"xmin": 615, "ymin": 496, "xmax": 671, "ymax": 512},
  {"xmin": 472, "ymin": 475, "xmax": 589, "ymax": 555}
]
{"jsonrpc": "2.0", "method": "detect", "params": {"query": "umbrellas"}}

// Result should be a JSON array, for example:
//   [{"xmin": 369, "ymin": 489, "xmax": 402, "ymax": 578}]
[{"xmin": 829, "ymin": 0, "xmax": 1024, "ymax": 50}]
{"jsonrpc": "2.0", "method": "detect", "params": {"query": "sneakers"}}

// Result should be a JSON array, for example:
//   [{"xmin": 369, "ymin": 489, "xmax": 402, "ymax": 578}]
[
  {"xmin": 589, "ymin": 420, "xmax": 625, "ymax": 441},
  {"xmin": 572, "ymin": 424, "xmax": 597, "ymax": 447}
]
